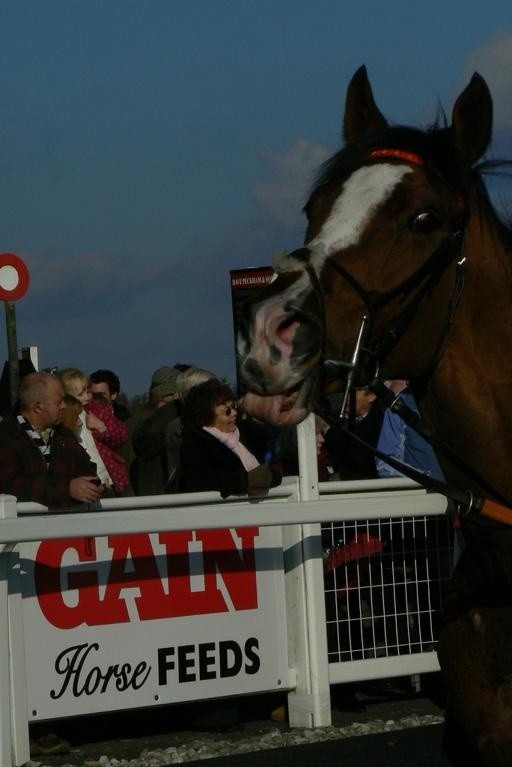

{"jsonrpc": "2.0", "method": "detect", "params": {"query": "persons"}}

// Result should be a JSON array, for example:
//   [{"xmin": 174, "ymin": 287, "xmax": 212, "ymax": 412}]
[{"xmin": 2, "ymin": 363, "xmax": 448, "ymax": 754}]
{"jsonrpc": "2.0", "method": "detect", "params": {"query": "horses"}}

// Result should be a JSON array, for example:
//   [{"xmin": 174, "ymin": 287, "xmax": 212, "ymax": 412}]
[{"xmin": 236, "ymin": 59, "xmax": 512, "ymax": 767}]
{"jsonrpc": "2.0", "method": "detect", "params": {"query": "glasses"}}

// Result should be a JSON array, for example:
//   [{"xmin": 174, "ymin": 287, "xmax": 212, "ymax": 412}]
[{"xmin": 218, "ymin": 402, "xmax": 236, "ymax": 416}]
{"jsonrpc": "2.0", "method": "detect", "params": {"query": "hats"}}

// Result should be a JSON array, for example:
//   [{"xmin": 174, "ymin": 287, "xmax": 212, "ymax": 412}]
[{"xmin": 150, "ymin": 367, "xmax": 182, "ymax": 403}]
